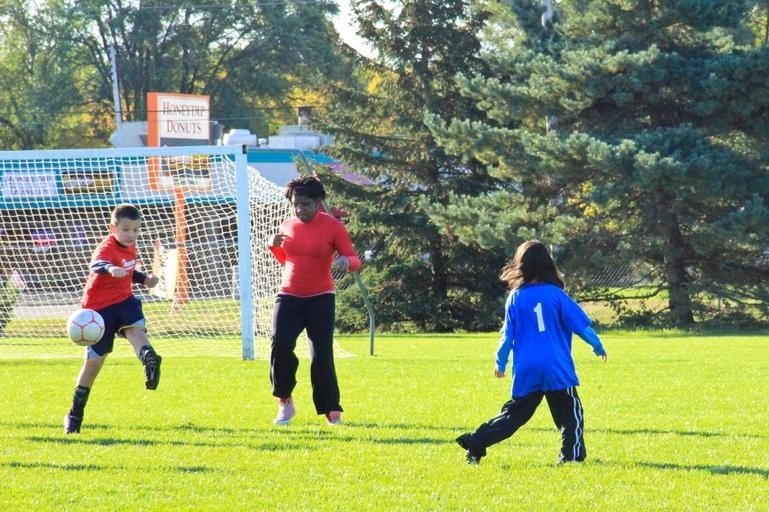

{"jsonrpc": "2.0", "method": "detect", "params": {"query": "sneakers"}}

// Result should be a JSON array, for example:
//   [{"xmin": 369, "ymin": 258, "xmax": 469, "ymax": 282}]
[
  {"xmin": 324, "ymin": 409, "xmax": 346, "ymax": 432},
  {"xmin": 141, "ymin": 352, "xmax": 161, "ymax": 393},
  {"xmin": 64, "ymin": 408, "xmax": 83, "ymax": 437},
  {"xmin": 274, "ymin": 397, "xmax": 295, "ymax": 425},
  {"xmin": 456, "ymin": 431, "xmax": 482, "ymax": 469}
]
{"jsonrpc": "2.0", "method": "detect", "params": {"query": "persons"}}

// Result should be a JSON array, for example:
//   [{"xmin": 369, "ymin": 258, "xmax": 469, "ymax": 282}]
[
  {"xmin": 454, "ymin": 240, "xmax": 607, "ymax": 468},
  {"xmin": 266, "ymin": 174, "xmax": 362, "ymax": 425},
  {"xmin": 63, "ymin": 204, "xmax": 162, "ymax": 433}
]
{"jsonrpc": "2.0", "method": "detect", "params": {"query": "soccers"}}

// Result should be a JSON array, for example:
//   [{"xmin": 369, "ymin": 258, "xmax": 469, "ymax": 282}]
[{"xmin": 68, "ymin": 309, "xmax": 105, "ymax": 347}]
